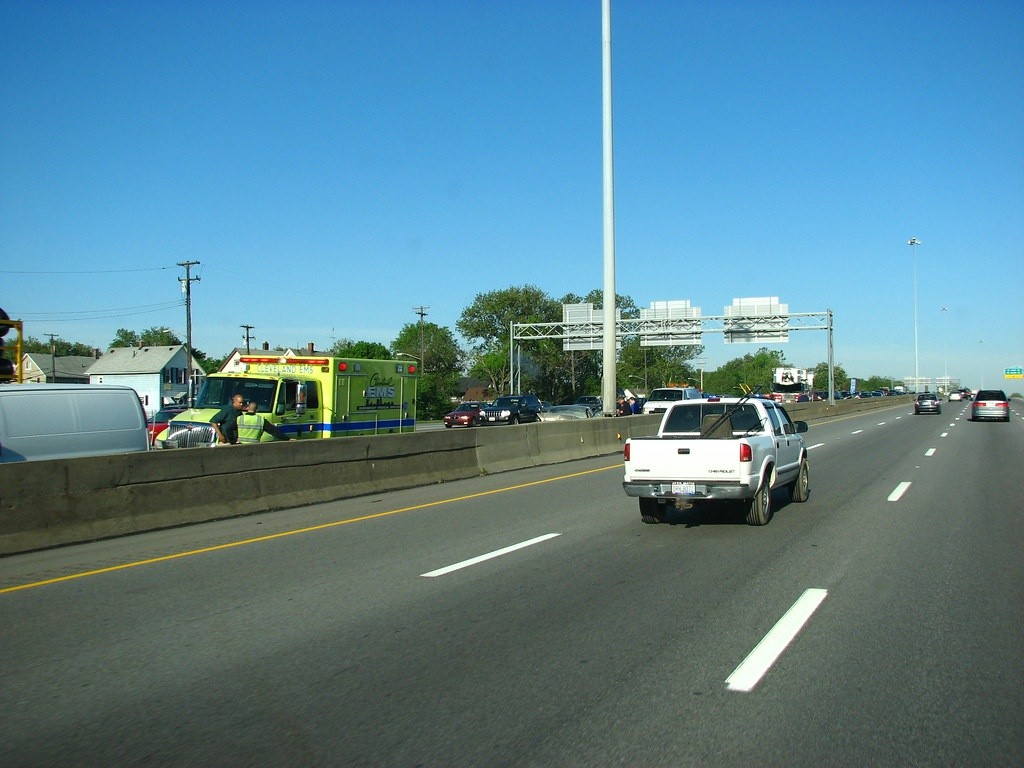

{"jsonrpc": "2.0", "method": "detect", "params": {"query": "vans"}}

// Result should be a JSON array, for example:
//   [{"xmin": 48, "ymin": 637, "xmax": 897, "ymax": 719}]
[{"xmin": 0, "ymin": 383, "xmax": 148, "ymax": 463}]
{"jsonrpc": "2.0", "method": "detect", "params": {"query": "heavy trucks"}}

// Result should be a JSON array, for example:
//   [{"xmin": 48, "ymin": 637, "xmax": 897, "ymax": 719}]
[{"xmin": 773, "ymin": 367, "xmax": 814, "ymax": 404}]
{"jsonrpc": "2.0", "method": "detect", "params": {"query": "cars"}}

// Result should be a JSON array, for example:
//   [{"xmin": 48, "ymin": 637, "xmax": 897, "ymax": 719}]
[
  {"xmin": 147, "ymin": 409, "xmax": 190, "ymax": 445},
  {"xmin": 958, "ymin": 389, "xmax": 976, "ymax": 400},
  {"xmin": 538, "ymin": 404, "xmax": 595, "ymax": 423},
  {"xmin": 948, "ymin": 391, "xmax": 962, "ymax": 401},
  {"xmin": 914, "ymin": 393, "xmax": 941, "ymax": 414},
  {"xmin": 575, "ymin": 396, "xmax": 603, "ymax": 413},
  {"xmin": 444, "ymin": 402, "xmax": 489, "ymax": 428},
  {"xmin": 793, "ymin": 385, "xmax": 907, "ymax": 403}
]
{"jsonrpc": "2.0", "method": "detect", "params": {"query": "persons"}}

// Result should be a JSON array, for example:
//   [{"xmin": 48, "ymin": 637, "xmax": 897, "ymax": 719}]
[
  {"xmin": 226, "ymin": 401, "xmax": 297, "ymax": 444},
  {"xmin": 618, "ymin": 397, "xmax": 633, "ymax": 416},
  {"xmin": 627, "ymin": 397, "xmax": 642, "ymax": 414},
  {"xmin": 209, "ymin": 394, "xmax": 245, "ymax": 444}
]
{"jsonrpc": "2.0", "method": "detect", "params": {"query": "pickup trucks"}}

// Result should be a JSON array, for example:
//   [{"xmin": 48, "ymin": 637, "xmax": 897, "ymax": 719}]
[{"xmin": 623, "ymin": 397, "xmax": 811, "ymax": 525}]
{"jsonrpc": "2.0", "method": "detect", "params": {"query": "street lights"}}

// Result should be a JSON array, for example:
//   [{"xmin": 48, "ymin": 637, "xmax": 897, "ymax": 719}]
[
  {"xmin": 939, "ymin": 306, "xmax": 947, "ymax": 396},
  {"xmin": 907, "ymin": 237, "xmax": 922, "ymax": 394}
]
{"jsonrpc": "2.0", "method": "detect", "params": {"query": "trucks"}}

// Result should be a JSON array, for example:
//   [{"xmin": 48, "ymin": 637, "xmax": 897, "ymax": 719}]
[{"xmin": 165, "ymin": 354, "xmax": 417, "ymax": 448}]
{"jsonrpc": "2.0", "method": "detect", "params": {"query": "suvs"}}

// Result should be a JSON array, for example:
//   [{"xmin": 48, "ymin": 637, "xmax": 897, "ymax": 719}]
[
  {"xmin": 642, "ymin": 388, "xmax": 701, "ymax": 415},
  {"xmin": 479, "ymin": 396, "xmax": 544, "ymax": 425},
  {"xmin": 972, "ymin": 390, "xmax": 1010, "ymax": 423}
]
